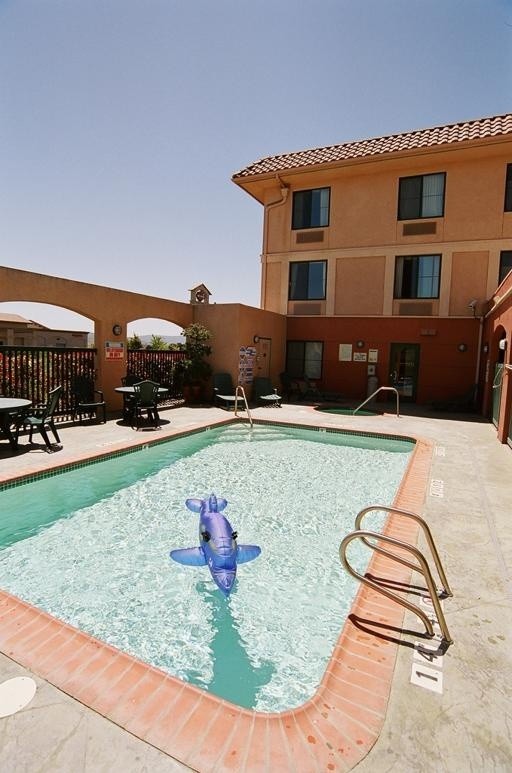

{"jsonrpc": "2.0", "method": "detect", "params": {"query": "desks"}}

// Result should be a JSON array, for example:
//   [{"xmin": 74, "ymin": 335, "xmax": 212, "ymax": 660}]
[{"xmin": 0, "ymin": 396, "xmax": 33, "ymax": 452}]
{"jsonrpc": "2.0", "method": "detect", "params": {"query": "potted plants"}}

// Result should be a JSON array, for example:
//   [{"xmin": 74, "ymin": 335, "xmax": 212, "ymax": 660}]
[{"xmin": 173, "ymin": 321, "xmax": 216, "ymax": 405}]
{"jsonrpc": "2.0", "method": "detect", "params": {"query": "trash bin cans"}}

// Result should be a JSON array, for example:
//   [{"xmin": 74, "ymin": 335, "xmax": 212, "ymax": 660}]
[{"xmin": 366, "ymin": 377, "xmax": 379, "ymax": 400}]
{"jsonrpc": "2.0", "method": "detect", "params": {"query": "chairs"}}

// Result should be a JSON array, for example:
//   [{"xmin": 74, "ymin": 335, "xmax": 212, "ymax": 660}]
[
  {"xmin": 13, "ymin": 383, "xmax": 65, "ymax": 453},
  {"xmin": 115, "ymin": 372, "xmax": 168, "ymax": 431},
  {"xmin": 213, "ymin": 372, "xmax": 245, "ymax": 411},
  {"xmin": 70, "ymin": 373, "xmax": 107, "ymax": 424},
  {"xmin": 253, "ymin": 376, "xmax": 282, "ymax": 407}
]
